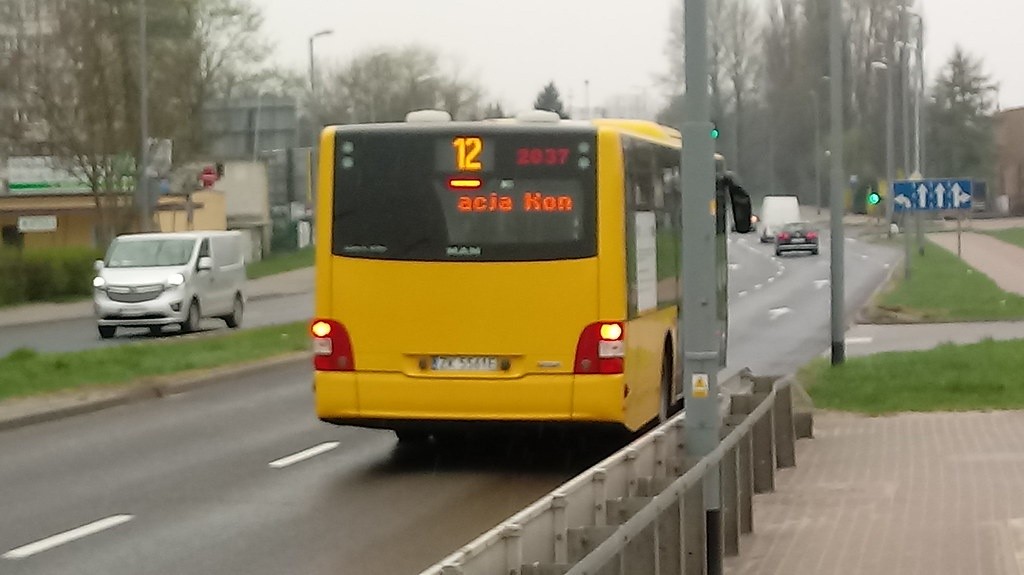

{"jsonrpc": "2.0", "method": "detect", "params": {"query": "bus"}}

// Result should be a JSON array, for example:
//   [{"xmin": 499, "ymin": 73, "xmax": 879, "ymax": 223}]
[{"xmin": 311, "ymin": 106, "xmax": 753, "ymax": 457}]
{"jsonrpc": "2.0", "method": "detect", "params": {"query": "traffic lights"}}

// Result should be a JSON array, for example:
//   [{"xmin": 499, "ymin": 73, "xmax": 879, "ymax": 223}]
[{"xmin": 868, "ymin": 192, "xmax": 882, "ymax": 205}]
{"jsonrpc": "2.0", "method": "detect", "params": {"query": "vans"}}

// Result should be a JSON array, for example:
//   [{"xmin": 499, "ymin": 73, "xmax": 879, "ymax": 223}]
[
  {"xmin": 757, "ymin": 195, "xmax": 799, "ymax": 242},
  {"xmin": 93, "ymin": 232, "xmax": 247, "ymax": 338}
]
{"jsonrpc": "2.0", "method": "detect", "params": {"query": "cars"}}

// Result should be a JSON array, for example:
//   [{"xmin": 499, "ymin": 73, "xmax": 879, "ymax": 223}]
[{"xmin": 774, "ymin": 223, "xmax": 819, "ymax": 257}]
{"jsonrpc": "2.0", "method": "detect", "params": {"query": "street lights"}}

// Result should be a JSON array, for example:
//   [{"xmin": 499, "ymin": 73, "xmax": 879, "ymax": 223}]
[
  {"xmin": 871, "ymin": 61, "xmax": 895, "ymax": 179},
  {"xmin": 309, "ymin": 29, "xmax": 332, "ymax": 98},
  {"xmin": 806, "ymin": 90, "xmax": 822, "ymax": 211},
  {"xmin": 899, "ymin": 5, "xmax": 923, "ymax": 175},
  {"xmin": 891, "ymin": 42, "xmax": 917, "ymax": 176}
]
